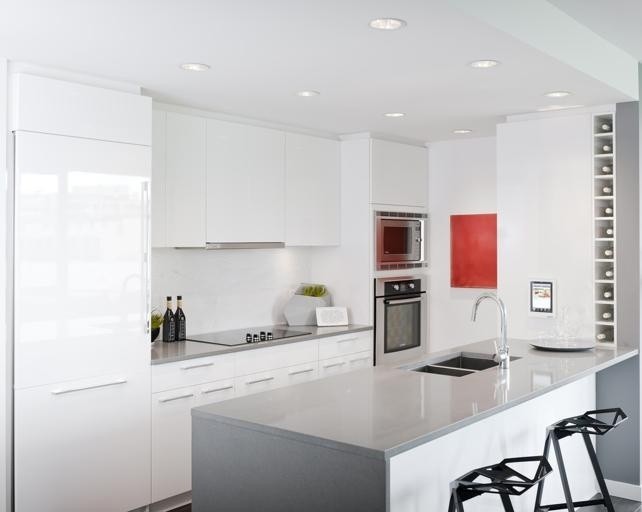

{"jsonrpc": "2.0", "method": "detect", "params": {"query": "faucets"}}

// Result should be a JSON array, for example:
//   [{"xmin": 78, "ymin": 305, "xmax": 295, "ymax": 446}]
[{"xmin": 471, "ymin": 291, "xmax": 511, "ymax": 369}]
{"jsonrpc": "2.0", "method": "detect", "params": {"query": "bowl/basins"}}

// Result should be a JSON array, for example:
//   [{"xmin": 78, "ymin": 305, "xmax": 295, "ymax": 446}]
[{"xmin": 151, "ymin": 327, "xmax": 160, "ymax": 342}]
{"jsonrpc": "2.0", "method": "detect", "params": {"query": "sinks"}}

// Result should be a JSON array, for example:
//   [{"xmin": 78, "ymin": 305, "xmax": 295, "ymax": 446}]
[
  {"xmin": 395, "ymin": 361, "xmax": 477, "ymax": 377},
  {"xmin": 426, "ymin": 351, "xmax": 522, "ymax": 370}
]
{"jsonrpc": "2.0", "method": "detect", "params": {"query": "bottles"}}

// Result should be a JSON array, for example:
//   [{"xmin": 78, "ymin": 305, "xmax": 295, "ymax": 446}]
[
  {"xmin": 175, "ymin": 295, "xmax": 186, "ymax": 340},
  {"xmin": 596, "ymin": 122, "xmax": 613, "ymax": 342},
  {"xmin": 164, "ymin": 296, "xmax": 176, "ymax": 341}
]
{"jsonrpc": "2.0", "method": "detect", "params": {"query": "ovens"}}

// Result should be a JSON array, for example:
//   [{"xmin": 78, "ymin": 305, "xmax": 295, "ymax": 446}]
[{"xmin": 375, "ymin": 278, "xmax": 430, "ymax": 370}]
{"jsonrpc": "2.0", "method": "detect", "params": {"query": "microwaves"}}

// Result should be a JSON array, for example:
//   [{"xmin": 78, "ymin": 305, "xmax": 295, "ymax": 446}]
[{"xmin": 376, "ymin": 217, "xmax": 425, "ymax": 263}]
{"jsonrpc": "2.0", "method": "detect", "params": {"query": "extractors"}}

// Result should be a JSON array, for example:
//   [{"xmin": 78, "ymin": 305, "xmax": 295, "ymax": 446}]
[{"xmin": 176, "ymin": 242, "xmax": 285, "ymax": 252}]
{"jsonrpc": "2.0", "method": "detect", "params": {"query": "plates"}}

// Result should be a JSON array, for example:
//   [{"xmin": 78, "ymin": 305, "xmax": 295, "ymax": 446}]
[{"xmin": 528, "ymin": 336, "xmax": 595, "ymax": 352}]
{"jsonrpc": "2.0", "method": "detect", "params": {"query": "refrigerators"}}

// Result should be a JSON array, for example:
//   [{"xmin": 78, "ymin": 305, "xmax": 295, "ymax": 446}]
[{"xmin": 11, "ymin": 130, "xmax": 155, "ymax": 511}]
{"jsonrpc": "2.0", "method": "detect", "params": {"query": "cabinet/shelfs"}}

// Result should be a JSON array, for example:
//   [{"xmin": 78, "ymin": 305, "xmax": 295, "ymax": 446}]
[
  {"xmin": 589, "ymin": 112, "xmax": 620, "ymax": 350},
  {"xmin": 153, "ymin": 105, "xmax": 206, "ymax": 250},
  {"xmin": 207, "ymin": 110, "xmax": 285, "ymax": 243},
  {"xmin": 152, "ymin": 352, "xmax": 238, "ymax": 511},
  {"xmin": 319, "ymin": 328, "xmax": 374, "ymax": 378},
  {"xmin": 238, "ymin": 336, "xmax": 318, "ymax": 399},
  {"xmin": 11, "ymin": 75, "xmax": 151, "ymax": 512},
  {"xmin": 367, "ymin": 137, "xmax": 430, "ymax": 210},
  {"xmin": 284, "ymin": 130, "xmax": 341, "ymax": 247}
]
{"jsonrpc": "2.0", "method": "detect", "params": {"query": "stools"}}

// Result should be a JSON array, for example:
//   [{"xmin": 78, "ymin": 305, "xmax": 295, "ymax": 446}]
[
  {"xmin": 532, "ymin": 402, "xmax": 632, "ymax": 512},
  {"xmin": 447, "ymin": 453, "xmax": 555, "ymax": 511}
]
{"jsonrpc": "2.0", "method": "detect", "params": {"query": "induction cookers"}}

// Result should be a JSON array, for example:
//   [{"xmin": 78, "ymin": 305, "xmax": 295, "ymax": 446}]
[{"xmin": 186, "ymin": 324, "xmax": 312, "ymax": 347}]
